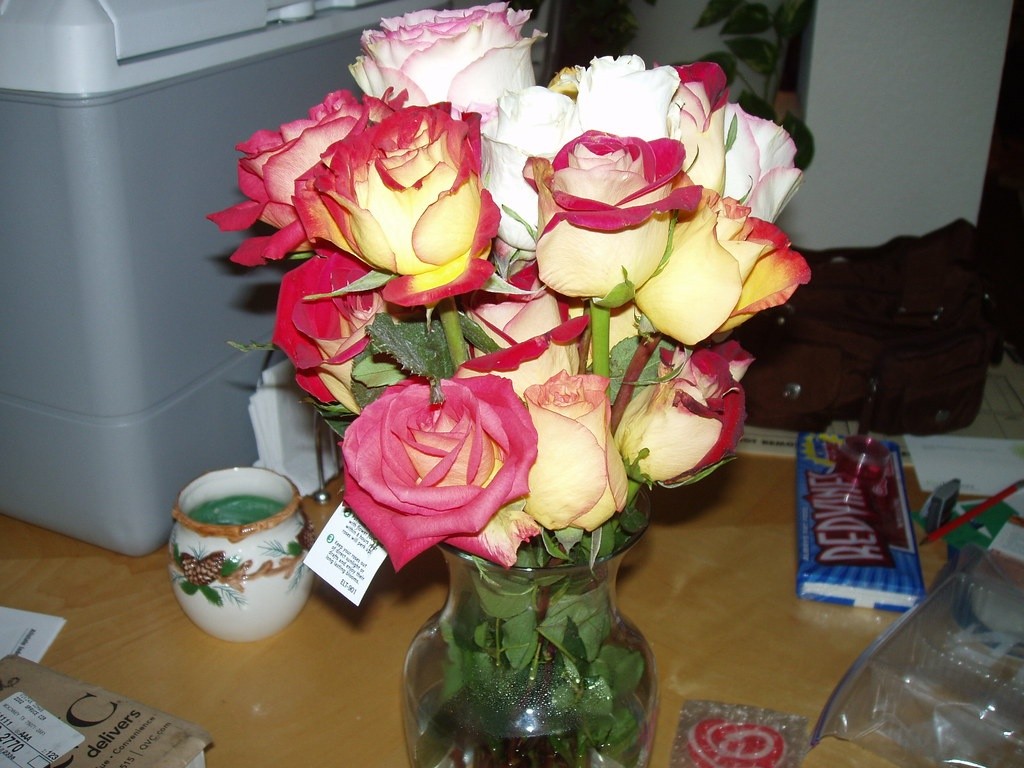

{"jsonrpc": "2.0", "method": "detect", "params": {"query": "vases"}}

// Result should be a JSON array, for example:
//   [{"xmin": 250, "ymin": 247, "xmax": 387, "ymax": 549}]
[{"xmin": 401, "ymin": 491, "xmax": 665, "ymax": 768}]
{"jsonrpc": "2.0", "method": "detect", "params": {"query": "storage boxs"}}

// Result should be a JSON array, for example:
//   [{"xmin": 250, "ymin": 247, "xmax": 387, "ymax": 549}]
[
  {"xmin": 0, "ymin": 655, "xmax": 212, "ymax": 768},
  {"xmin": 0, "ymin": 0, "xmax": 452, "ymax": 556},
  {"xmin": 796, "ymin": 430, "xmax": 926, "ymax": 613}
]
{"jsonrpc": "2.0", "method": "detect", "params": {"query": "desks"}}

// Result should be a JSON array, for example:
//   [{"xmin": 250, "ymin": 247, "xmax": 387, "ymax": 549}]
[{"xmin": 0, "ymin": 450, "xmax": 1024, "ymax": 768}]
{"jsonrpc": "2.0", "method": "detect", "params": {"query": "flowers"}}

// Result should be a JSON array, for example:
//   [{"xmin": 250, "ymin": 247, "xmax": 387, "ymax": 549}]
[{"xmin": 204, "ymin": 2, "xmax": 814, "ymax": 572}]
